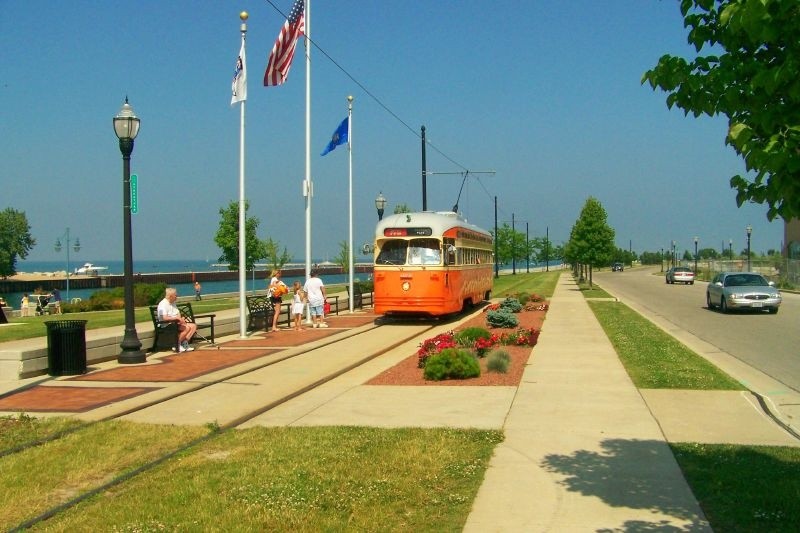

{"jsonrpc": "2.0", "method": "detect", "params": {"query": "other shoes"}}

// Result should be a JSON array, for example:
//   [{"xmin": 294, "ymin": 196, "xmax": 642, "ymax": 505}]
[
  {"xmin": 272, "ymin": 328, "xmax": 279, "ymax": 332},
  {"xmin": 319, "ymin": 324, "xmax": 328, "ymax": 328},
  {"xmin": 313, "ymin": 324, "xmax": 318, "ymax": 328},
  {"xmin": 182, "ymin": 343, "xmax": 194, "ymax": 351},
  {"xmin": 172, "ymin": 345, "xmax": 186, "ymax": 352}
]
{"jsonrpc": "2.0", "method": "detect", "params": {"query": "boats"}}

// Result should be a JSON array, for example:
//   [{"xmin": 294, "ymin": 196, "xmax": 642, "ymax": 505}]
[{"xmin": 76, "ymin": 263, "xmax": 108, "ymax": 272}]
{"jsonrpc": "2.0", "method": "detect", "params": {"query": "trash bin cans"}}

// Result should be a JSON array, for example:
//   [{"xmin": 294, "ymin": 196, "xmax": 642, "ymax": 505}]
[{"xmin": 44, "ymin": 319, "xmax": 89, "ymax": 375}]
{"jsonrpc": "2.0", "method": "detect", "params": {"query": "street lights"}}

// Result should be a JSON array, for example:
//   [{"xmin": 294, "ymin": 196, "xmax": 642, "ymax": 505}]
[
  {"xmin": 375, "ymin": 192, "xmax": 386, "ymax": 220},
  {"xmin": 54, "ymin": 227, "xmax": 82, "ymax": 303},
  {"xmin": 115, "ymin": 93, "xmax": 148, "ymax": 364},
  {"xmin": 693, "ymin": 236, "xmax": 699, "ymax": 277},
  {"xmin": 672, "ymin": 239, "xmax": 676, "ymax": 268},
  {"xmin": 728, "ymin": 239, "xmax": 734, "ymax": 260},
  {"xmin": 745, "ymin": 224, "xmax": 752, "ymax": 273}
]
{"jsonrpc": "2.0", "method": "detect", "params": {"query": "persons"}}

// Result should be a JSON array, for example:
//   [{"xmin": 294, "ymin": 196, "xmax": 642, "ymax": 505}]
[
  {"xmin": 21, "ymin": 294, "xmax": 29, "ymax": 320},
  {"xmin": 158, "ymin": 288, "xmax": 196, "ymax": 352},
  {"xmin": 51, "ymin": 287, "xmax": 61, "ymax": 314},
  {"xmin": 194, "ymin": 282, "xmax": 201, "ymax": 301},
  {"xmin": 269, "ymin": 268, "xmax": 328, "ymax": 333}
]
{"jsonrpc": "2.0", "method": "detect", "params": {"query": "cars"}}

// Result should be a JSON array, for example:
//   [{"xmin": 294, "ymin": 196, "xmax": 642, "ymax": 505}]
[
  {"xmin": 707, "ymin": 270, "xmax": 782, "ymax": 315},
  {"xmin": 612, "ymin": 261, "xmax": 624, "ymax": 271},
  {"xmin": 666, "ymin": 267, "xmax": 695, "ymax": 286}
]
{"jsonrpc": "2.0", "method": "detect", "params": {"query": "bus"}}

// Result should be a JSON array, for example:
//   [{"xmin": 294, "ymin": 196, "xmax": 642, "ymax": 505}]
[{"xmin": 372, "ymin": 211, "xmax": 496, "ymax": 321}]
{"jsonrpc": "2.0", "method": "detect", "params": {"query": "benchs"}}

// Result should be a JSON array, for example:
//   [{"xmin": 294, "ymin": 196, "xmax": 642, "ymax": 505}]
[
  {"xmin": 346, "ymin": 285, "xmax": 372, "ymax": 309},
  {"xmin": 149, "ymin": 303, "xmax": 216, "ymax": 352},
  {"xmin": 246, "ymin": 295, "xmax": 292, "ymax": 332}
]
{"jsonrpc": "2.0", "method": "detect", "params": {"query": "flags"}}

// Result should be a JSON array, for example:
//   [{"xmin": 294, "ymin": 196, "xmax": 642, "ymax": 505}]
[
  {"xmin": 321, "ymin": 116, "xmax": 350, "ymax": 156},
  {"xmin": 231, "ymin": 39, "xmax": 246, "ymax": 104},
  {"xmin": 263, "ymin": 0, "xmax": 305, "ymax": 86}
]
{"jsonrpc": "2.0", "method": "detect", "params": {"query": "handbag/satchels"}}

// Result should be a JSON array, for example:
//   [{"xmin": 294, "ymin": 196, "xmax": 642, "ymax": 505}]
[{"xmin": 324, "ymin": 300, "xmax": 330, "ymax": 315}]
